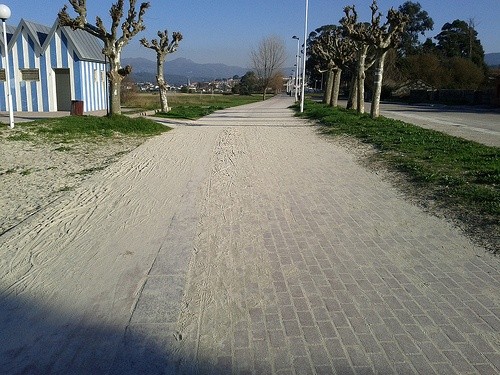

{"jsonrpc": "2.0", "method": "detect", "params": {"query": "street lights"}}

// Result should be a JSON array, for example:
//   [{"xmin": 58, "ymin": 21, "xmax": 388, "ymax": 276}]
[
  {"xmin": 287, "ymin": 33, "xmax": 305, "ymax": 113},
  {"xmin": 0, "ymin": 3, "xmax": 15, "ymax": 130}
]
